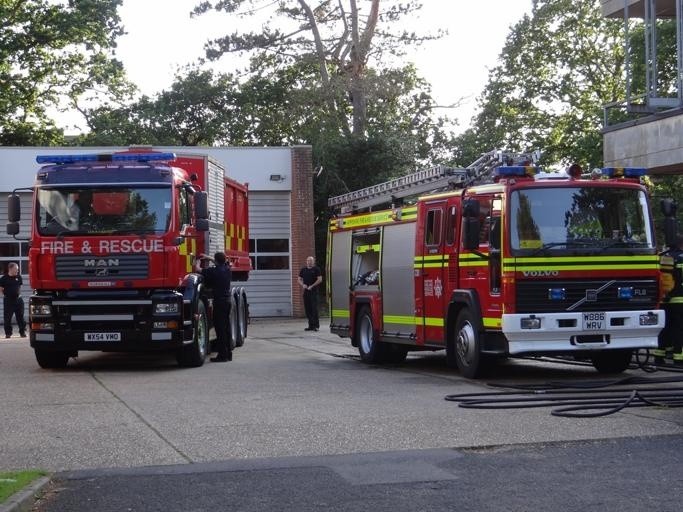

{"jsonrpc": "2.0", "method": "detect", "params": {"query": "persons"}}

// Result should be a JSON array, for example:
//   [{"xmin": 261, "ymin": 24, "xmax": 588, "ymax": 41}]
[
  {"xmin": 653, "ymin": 241, "xmax": 683, "ymax": 367},
  {"xmin": 0, "ymin": 261, "xmax": 27, "ymax": 338},
  {"xmin": 298, "ymin": 255, "xmax": 322, "ymax": 331},
  {"xmin": 194, "ymin": 252, "xmax": 232, "ymax": 363}
]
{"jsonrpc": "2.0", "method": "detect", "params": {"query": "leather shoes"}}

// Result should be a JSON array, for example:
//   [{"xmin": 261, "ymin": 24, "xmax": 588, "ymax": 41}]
[
  {"xmin": 305, "ymin": 328, "xmax": 320, "ymax": 332},
  {"xmin": 210, "ymin": 356, "xmax": 232, "ymax": 363}
]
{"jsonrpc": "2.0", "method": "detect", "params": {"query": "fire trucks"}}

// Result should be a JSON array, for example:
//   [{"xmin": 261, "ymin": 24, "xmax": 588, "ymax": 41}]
[
  {"xmin": 5, "ymin": 143, "xmax": 255, "ymax": 371},
  {"xmin": 322, "ymin": 144, "xmax": 681, "ymax": 382}
]
